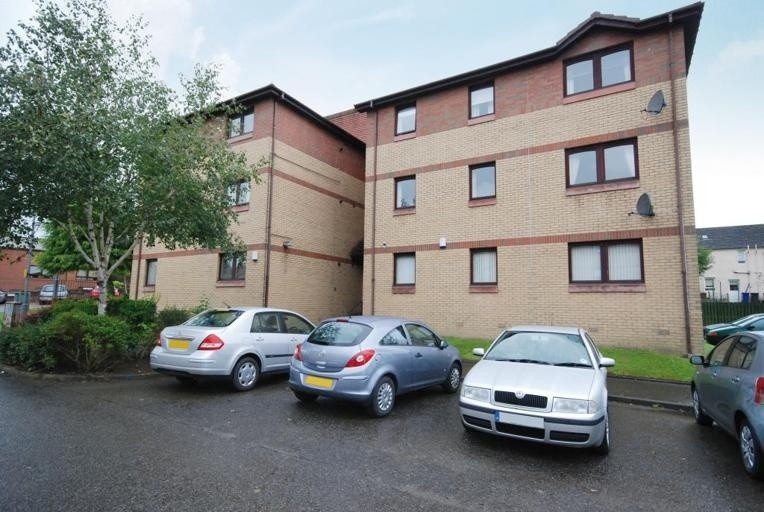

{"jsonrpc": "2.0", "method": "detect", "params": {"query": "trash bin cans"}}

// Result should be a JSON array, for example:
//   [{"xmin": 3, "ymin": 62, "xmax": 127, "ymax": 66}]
[
  {"xmin": 742, "ymin": 293, "xmax": 749, "ymax": 302},
  {"xmin": 751, "ymin": 293, "xmax": 758, "ymax": 303},
  {"xmin": 15, "ymin": 292, "xmax": 30, "ymax": 303}
]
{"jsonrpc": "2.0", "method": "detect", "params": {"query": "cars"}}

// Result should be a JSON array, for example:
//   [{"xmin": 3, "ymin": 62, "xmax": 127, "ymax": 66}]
[
  {"xmin": 287, "ymin": 314, "xmax": 463, "ymax": 418},
  {"xmin": 39, "ymin": 284, "xmax": 71, "ymax": 305},
  {"xmin": 91, "ymin": 285, "xmax": 120, "ymax": 299},
  {"xmin": 149, "ymin": 307, "xmax": 317, "ymax": 392},
  {"xmin": 689, "ymin": 313, "xmax": 764, "ymax": 478},
  {"xmin": 0, "ymin": 290, "xmax": 7, "ymax": 304},
  {"xmin": 459, "ymin": 325, "xmax": 615, "ymax": 455}
]
